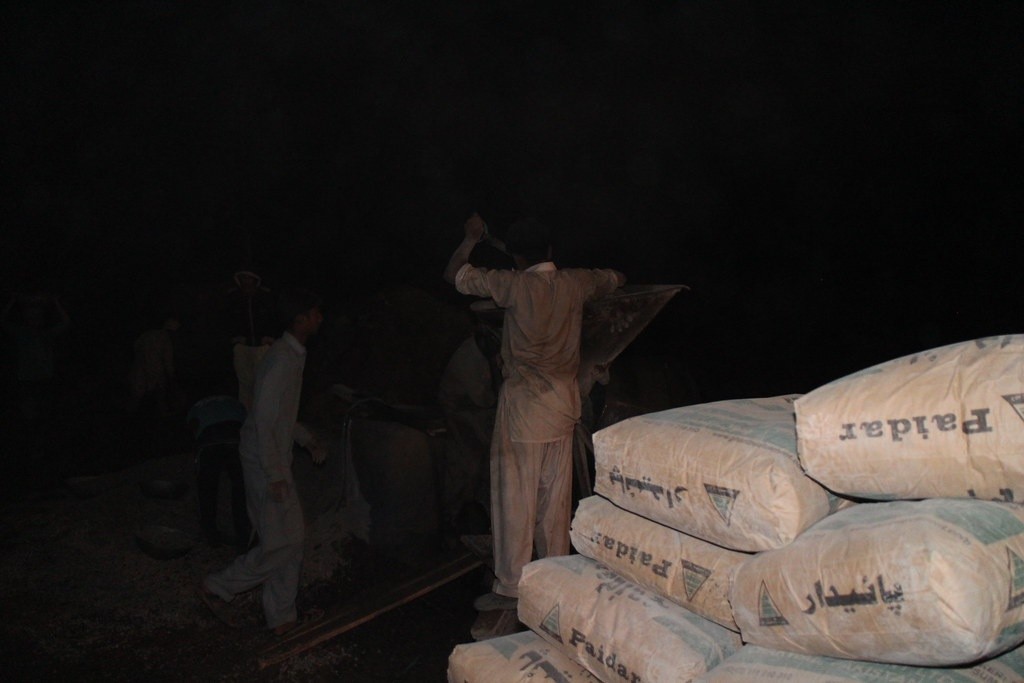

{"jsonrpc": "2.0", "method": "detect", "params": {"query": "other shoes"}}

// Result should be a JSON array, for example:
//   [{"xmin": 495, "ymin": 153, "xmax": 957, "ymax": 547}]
[{"xmin": 474, "ymin": 592, "xmax": 519, "ymax": 611}]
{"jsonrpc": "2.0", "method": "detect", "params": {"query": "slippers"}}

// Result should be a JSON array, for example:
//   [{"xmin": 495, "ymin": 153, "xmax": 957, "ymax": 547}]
[
  {"xmin": 271, "ymin": 606, "xmax": 326, "ymax": 642},
  {"xmin": 197, "ymin": 586, "xmax": 246, "ymax": 629}
]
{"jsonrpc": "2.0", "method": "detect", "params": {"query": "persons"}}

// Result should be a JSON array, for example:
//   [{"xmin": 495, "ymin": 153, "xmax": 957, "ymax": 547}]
[
  {"xmin": 186, "ymin": 288, "xmax": 331, "ymax": 635},
  {"xmin": 444, "ymin": 212, "xmax": 628, "ymax": 611}
]
